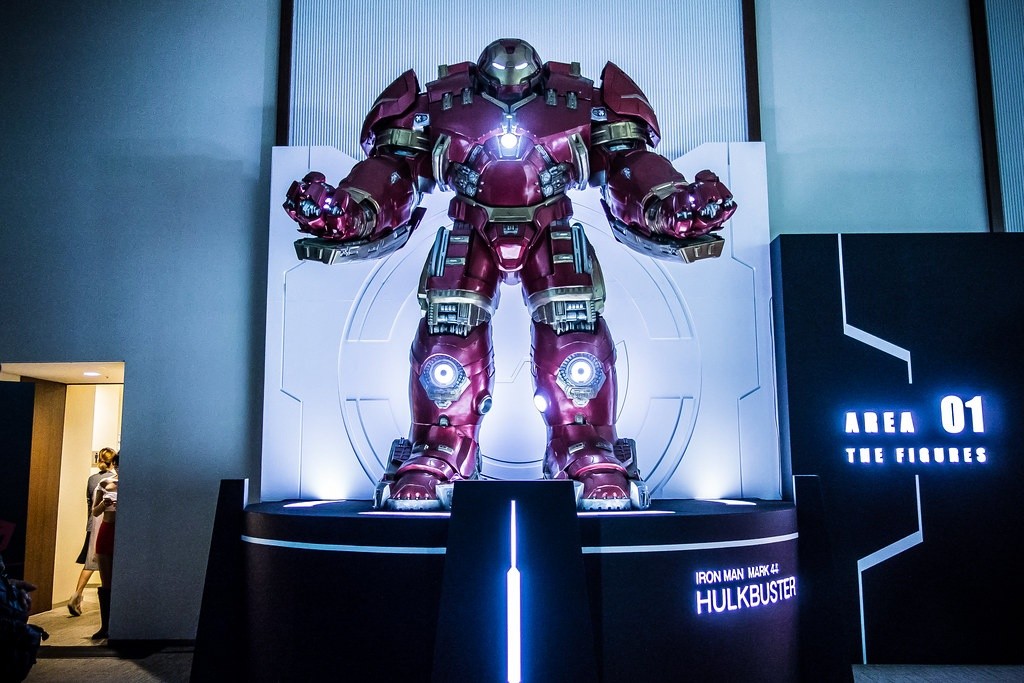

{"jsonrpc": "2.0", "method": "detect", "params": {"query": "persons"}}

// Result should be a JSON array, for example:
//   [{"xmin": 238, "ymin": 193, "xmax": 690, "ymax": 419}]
[
  {"xmin": 282, "ymin": 38, "xmax": 738, "ymax": 512},
  {"xmin": 67, "ymin": 447, "xmax": 116, "ymax": 616},
  {"xmin": 90, "ymin": 450, "xmax": 120, "ymax": 639}
]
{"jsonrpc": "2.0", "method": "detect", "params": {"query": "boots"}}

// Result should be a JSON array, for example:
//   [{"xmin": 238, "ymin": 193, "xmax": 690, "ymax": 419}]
[{"xmin": 91, "ymin": 587, "xmax": 111, "ymax": 640}]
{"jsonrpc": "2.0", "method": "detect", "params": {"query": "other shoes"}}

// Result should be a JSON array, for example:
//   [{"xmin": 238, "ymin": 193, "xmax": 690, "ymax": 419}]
[{"xmin": 67, "ymin": 591, "xmax": 81, "ymax": 616}]
